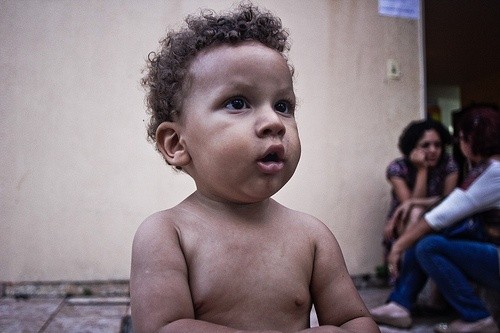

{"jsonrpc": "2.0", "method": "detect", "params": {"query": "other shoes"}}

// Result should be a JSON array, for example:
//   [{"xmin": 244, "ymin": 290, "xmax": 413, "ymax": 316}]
[
  {"xmin": 369, "ymin": 310, "xmax": 411, "ymax": 330},
  {"xmin": 433, "ymin": 318, "xmax": 496, "ymax": 332}
]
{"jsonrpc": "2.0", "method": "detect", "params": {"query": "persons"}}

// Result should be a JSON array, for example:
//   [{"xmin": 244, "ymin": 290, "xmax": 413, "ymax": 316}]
[
  {"xmin": 381, "ymin": 118, "xmax": 459, "ymax": 313},
  {"xmin": 367, "ymin": 107, "xmax": 500, "ymax": 333},
  {"xmin": 129, "ymin": 0, "xmax": 381, "ymax": 333}
]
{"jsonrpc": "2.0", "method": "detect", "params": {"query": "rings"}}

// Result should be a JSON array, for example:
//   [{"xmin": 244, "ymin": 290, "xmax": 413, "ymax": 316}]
[{"xmin": 388, "ymin": 264, "xmax": 393, "ymax": 272}]
